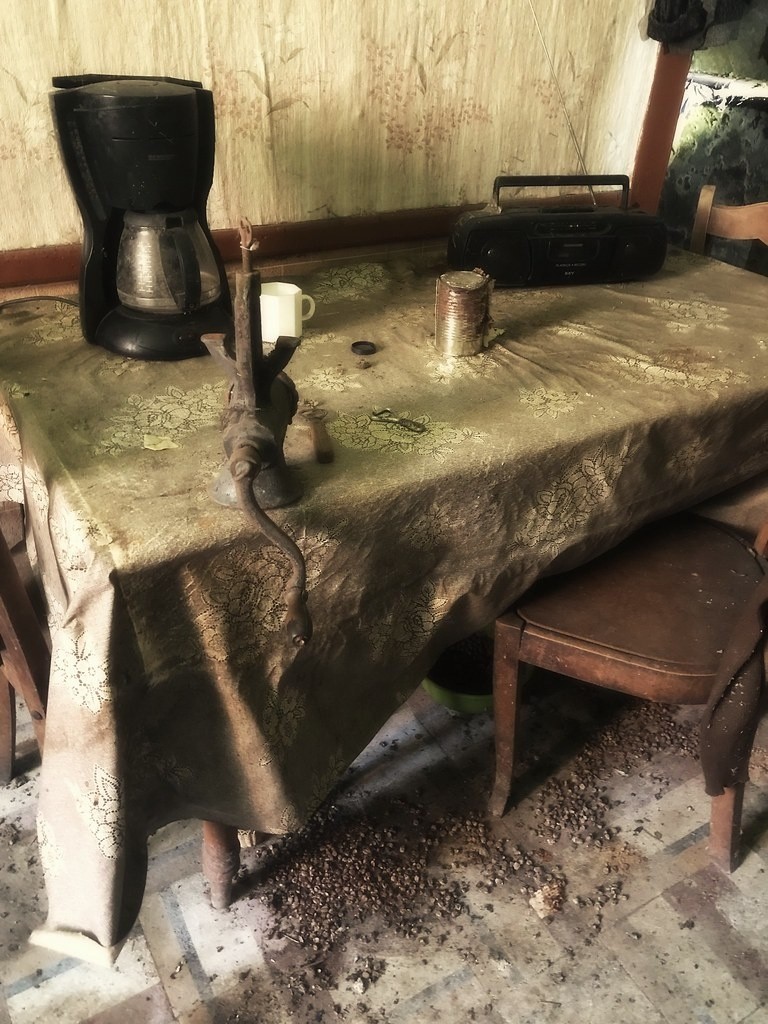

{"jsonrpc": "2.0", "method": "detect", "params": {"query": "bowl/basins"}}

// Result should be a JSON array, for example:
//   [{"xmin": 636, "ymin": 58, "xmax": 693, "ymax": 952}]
[{"xmin": 421, "ymin": 662, "xmax": 532, "ymax": 715}]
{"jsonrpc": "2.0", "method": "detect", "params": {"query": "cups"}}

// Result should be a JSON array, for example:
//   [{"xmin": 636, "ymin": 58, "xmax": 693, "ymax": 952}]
[{"xmin": 257, "ymin": 281, "xmax": 315, "ymax": 342}]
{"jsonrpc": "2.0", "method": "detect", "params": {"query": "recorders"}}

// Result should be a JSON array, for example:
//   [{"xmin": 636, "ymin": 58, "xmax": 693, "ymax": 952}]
[{"xmin": 447, "ymin": 174, "xmax": 669, "ymax": 290}]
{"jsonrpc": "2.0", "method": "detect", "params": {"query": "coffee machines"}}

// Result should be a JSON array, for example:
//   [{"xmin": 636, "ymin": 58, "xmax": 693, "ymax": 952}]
[{"xmin": 47, "ymin": 74, "xmax": 233, "ymax": 363}]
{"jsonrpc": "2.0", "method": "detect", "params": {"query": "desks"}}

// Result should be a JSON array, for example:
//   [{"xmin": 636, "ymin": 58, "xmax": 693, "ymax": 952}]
[{"xmin": 1, "ymin": 234, "xmax": 767, "ymax": 972}]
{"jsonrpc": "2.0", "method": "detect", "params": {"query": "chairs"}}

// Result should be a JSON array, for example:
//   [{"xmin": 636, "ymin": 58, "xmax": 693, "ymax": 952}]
[
  {"xmin": 687, "ymin": 182, "xmax": 768, "ymax": 257},
  {"xmin": 489, "ymin": 517, "xmax": 768, "ymax": 876}
]
